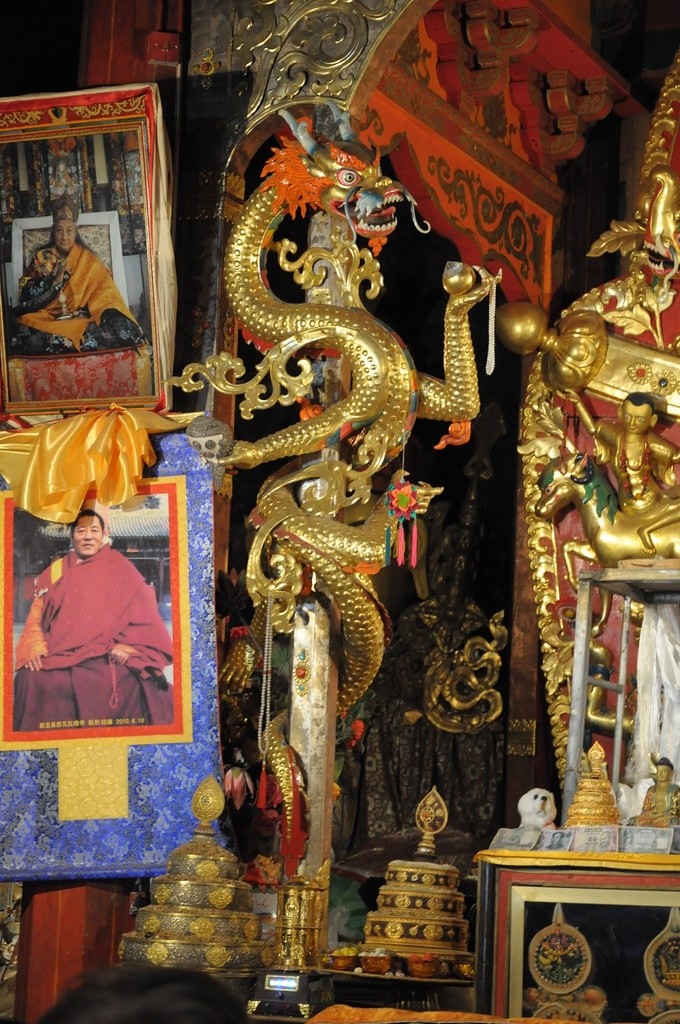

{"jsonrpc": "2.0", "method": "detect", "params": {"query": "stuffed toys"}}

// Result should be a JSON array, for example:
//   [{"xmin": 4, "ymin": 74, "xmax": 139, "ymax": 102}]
[{"xmin": 519, "ymin": 788, "xmax": 559, "ymax": 831}]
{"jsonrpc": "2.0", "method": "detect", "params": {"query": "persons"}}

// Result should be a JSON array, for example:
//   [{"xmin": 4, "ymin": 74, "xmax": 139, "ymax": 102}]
[
  {"xmin": 642, "ymin": 758, "xmax": 680, "ymax": 826},
  {"xmin": 13, "ymin": 195, "xmax": 142, "ymax": 360},
  {"xmin": 594, "ymin": 391, "xmax": 678, "ymax": 513},
  {"xmin": 18, "ymin": 504, "xmax": 176, "ymax": 732},
  {"xmin": 639, "ymin": 508, "xmax": 680, "ymax": 554}
]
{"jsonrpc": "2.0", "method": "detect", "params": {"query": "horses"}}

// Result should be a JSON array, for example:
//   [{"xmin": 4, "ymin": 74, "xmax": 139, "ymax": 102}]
[{"xmin": 532, "ymin": 451, "xmax": 680, "ymax": 628}]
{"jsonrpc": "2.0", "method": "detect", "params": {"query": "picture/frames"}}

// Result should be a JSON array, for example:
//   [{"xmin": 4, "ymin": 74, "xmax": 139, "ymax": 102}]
[
  {"xmin": 472, "ymin": 849, "xmax": 680, "ymax": 1024},
  {"xmin": 0, "ymin": 82, "xmax": 177, "ymax": 420}
]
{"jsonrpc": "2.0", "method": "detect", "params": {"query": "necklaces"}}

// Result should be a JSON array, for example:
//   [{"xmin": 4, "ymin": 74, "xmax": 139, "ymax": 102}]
[{"xmin": 623, "ymin": 433, "xmax": 650, "ymax": 500}]
